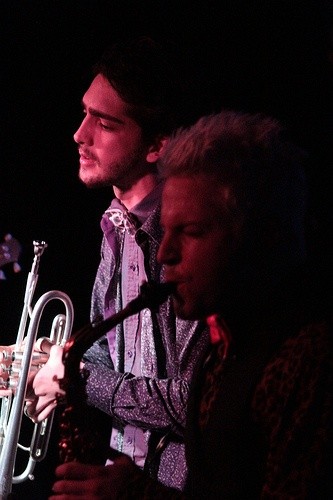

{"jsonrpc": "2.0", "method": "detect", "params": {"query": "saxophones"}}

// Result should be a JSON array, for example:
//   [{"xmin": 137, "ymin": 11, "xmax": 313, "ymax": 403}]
[{"xmin": 57, "ymin": 280, "xmax": 176, "ymax": 465}]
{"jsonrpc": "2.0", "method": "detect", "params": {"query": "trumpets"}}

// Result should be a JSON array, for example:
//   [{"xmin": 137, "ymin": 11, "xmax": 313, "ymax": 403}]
[{"xmin": 0, "ymin": 241, "xmax": 74, "ymax": 500}]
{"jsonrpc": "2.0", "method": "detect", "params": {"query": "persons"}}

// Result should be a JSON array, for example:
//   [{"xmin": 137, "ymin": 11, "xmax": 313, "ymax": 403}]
[
  {"xmin": 1, "ymin": 53, "xmax": 209, "ymax": 492},
  {"xmin": 47, "ymin": 111, "xmax": 333, "ymax": 500}
]
